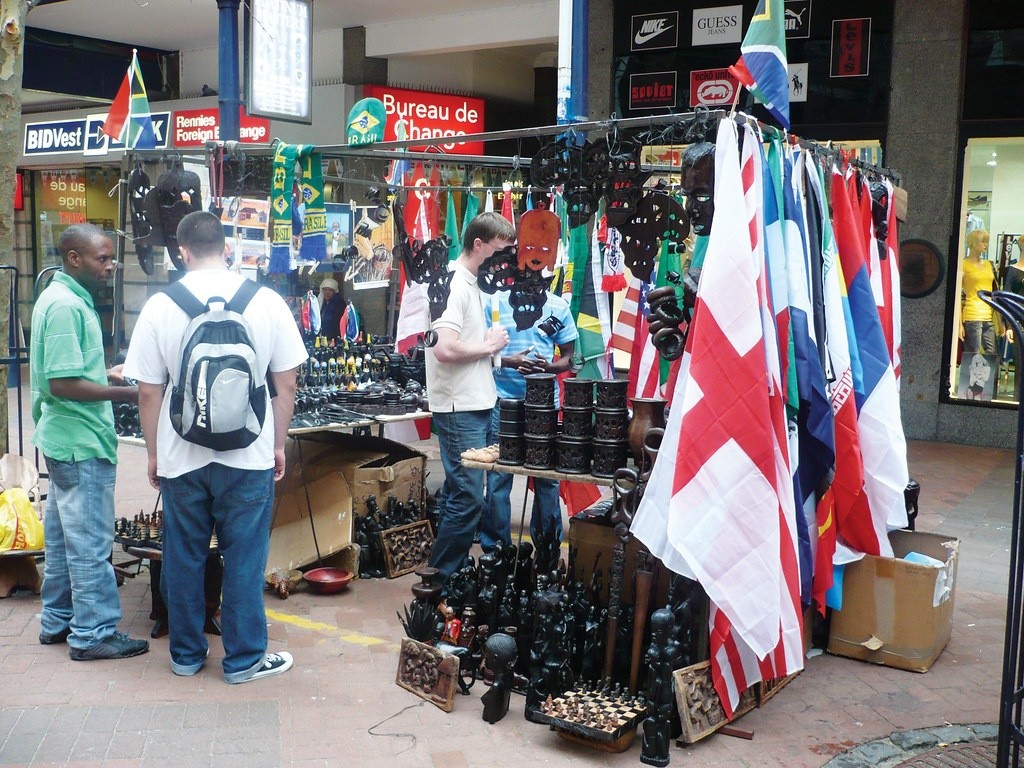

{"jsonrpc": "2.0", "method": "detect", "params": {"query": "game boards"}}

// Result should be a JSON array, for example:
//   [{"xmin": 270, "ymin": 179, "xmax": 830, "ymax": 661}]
[{"xmin": 528, "ymin": 682, "xmax": 648, "ymax": 734}]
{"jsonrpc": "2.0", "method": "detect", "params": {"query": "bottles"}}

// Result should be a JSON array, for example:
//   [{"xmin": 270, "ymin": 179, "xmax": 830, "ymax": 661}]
[{"xmin": 301, "ymin": 332, "xmax": 374, "ymax": 385}]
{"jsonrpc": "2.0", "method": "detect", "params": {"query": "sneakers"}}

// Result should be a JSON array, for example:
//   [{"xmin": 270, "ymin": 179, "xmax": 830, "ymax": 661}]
[
  {"xmin": 39, "ymin": 627, "xmax": 73, "ymax": 644},
  {"xmin": 70, "ymin": 630, "xmax": 150, "ymax": 661},
  {"xmin": 238, "ymin": 652, "xmax": 293, "ymax": 683}
]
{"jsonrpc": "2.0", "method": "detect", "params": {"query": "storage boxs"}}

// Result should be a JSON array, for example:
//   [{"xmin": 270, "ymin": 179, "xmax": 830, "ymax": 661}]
[
  {"xmin": 262, "ymin": 461, "xmax": 353, "ymax": 579},
  {"xmin": 275, "ymin": 430, "xmax": 427, "ymax": 517},
  {"xmin": 825, "ymin": 531, "xmax": 964, "ymax": 673}
]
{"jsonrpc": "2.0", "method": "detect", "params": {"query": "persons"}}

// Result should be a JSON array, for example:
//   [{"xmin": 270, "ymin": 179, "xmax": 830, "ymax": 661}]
[
  {"xmin": 123, "ymin": 210, "xmax": 307, "ymax": 684},
  {"xmin": 425, "ymin": 213, "xmax": 517, "ymax": 592},
  {"xmin": 959, "ymin": 230, "xmax": 1006, "ymax": 354},
  {"xmin": 29, "ymin": 224, "xmax": 150, "ymax": 661},
  {"xmin": 357, "ymin": 483, "xmax": 691, "ymax": 767},
  {"xmin": 484, "ymin": 275, "xmax": 579, "ymax": 560},
  {"xmin": 1005, "ymin": 234, "xmax": 1024, "ymax": 403},
  {"xmin": 318, "ymin": 279, "xmax": 346, "ymax": 342}
]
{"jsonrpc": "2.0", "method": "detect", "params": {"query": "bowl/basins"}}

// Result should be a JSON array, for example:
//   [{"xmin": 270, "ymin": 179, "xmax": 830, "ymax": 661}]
[
  {"xmin": 266, "ymin": 570, "xmax": 302, "ymax": 591},
  {"xmin": 336, "ymin": 391, "xmax": 400, "ymax": 404},
  {"xmin": 303, "ymin": 567, "xmax": 354, "ymax": 593}
]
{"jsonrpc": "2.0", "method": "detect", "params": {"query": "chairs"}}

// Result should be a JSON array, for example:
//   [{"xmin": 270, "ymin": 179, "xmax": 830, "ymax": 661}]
[{"xmin": 978, "ymin": 289, "xmax": 1023, "ymax": 404}]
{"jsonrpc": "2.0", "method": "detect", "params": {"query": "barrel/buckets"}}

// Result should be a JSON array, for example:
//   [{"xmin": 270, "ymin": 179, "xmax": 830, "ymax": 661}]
[{"xmin": 413, "ymin": 418, "xmax": 431, "ymax": 439}]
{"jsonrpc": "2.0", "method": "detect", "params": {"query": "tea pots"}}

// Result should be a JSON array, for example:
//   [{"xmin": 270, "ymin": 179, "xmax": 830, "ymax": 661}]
[
  {"xmin": 401, "ymin": 379, "xmax": 429, "ymax": 412},
  {"xmin": 366, "ymin": 378, "xmax": 404, "ymax": 394}
]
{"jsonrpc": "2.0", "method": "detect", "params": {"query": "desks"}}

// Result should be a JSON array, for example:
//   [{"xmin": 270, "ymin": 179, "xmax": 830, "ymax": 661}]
[
  {"xmin": 114, "ymin": 408, "xmax": 436, "ymax": 572},
  {"xmin": 461, "ymin": 457, "xmax": 640, "ymax": 588}
]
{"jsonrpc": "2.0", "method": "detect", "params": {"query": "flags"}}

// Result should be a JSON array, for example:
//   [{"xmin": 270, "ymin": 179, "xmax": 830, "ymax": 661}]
[
  {"xmin": 729, "ymin": 0, "xmax": 790, "ymax": 130},
  {"xmin": 100, "ymin": 52, "xmax": 909, "ymax": 722}
]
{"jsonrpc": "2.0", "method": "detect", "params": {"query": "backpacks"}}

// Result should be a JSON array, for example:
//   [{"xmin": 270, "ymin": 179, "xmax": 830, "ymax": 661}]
[{"xmin": 159, "ymin": 277, "xmax": 268, "ymax": 451}]
